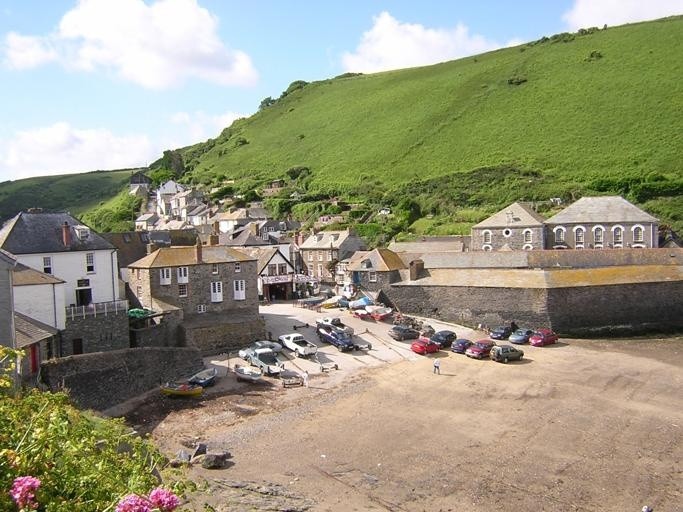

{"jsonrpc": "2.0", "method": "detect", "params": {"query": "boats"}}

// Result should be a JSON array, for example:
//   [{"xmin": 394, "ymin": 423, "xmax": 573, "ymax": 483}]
[
  {"xmin": 189, "ymin": 366, "xmax": 218, "ymax": 387},
  {"xmin": 159, "ymin": 379, "xmax": 205, "ymax": 399},
  {"xmin": 297, "ymin": 293, "xmax": 393, "ymax": 324},
  {"xmin": 233, "ymin": 366, "xmax": 261, "ymax": 383}
]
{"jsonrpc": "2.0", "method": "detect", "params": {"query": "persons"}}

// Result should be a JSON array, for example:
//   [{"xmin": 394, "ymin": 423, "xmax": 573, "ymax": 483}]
[{"xmin": 433, "ymin": 355, "xmax": 441, "ymax": 375}]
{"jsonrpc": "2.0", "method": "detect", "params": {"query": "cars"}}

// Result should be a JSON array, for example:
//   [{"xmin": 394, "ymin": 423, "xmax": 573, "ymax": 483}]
[
  {"xmin": 384, "ymin": 324, "xmax": 561, "ymax": 364},
  {"xmin": 238, "ymin": 341, "xmax": 283, "ymax": 362}
]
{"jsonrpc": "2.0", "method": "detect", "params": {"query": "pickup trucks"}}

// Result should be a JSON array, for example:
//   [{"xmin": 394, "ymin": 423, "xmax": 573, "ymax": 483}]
[
  {"xmin": 316, "ymin": 326, "xmax": 355, "ymax": 353},
  {"xmin": 315, "ymin": 316, "xmax": 356, "ymax": 338},
  {"xmin": 277, "ymin": 333, "xmax": 319, "ymax": 360}
]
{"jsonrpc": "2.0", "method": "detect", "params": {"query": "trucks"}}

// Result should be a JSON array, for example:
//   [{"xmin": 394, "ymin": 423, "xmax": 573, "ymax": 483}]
[{"xmin": 247, "ymin": 348, "xmax": 287, "ymax": 378}]
